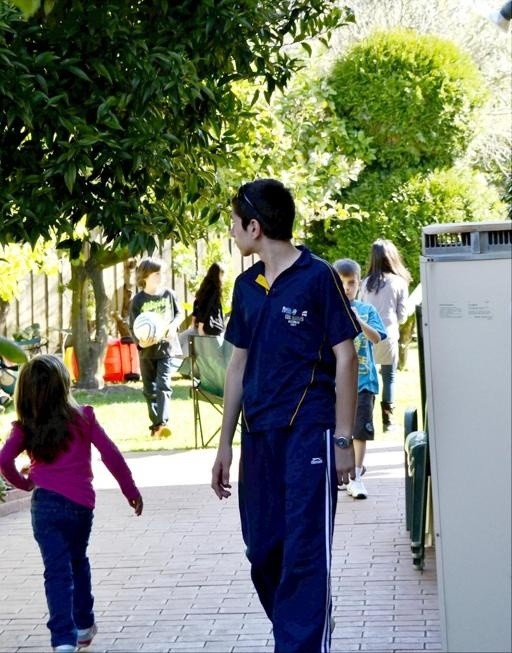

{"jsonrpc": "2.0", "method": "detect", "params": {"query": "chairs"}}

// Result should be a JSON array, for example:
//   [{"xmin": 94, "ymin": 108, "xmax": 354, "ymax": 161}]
[{"xmin": 187, "ymin": 335, "xmax": 242, "ymax": 448}]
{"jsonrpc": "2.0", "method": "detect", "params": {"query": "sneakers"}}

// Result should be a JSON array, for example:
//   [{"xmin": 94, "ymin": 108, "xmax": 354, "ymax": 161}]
[
  {"xmin": 54, "ymin": 624, "xmax": 97, "ymax": 652},
  {"xmin": 338, "ymin": 463, "xmax": 368, "ymax": 499},
  {"xmin": 151, "ymin": 425, "xmax": 170, "ymax": 439}
]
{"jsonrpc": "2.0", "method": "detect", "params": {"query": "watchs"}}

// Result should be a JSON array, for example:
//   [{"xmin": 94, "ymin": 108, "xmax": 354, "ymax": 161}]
[{"xmin": 332, "ymin": 436, "xmax": 355, "ymax": 448}]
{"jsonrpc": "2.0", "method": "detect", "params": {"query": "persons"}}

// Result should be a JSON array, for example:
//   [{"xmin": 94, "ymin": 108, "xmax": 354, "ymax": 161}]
[
  {"xmin": 128, "ymin": 259, "xmax": 186, "ymax": 438},
  {"xmin": 0, "ymin": 357, "xmax": 146, "ymax": 652},
  {"xmin": 211, "ymin": 178, "xmax": 360, "ymax": 653},
  {"xmin": 357, "ymin": 237, "xmax": 411, "ymax": 433},
  {"xmin": 331, "ymin": 259, "xmax": 387, "ymax": 500},
  {"xmin": 109, "ymin": 260, "xmax": 142, "ymax": 337},
  {"xmin": 185, "ymin": 262, "xmax": 224, "ymax": 379},
  {"xmin": 1, "ymin": 297, "xmax": 21, "ymax": 501}
]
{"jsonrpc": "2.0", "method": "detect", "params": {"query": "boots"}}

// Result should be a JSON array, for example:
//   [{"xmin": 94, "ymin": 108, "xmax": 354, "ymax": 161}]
[{"xmin": 381, "ymin": 401, "xmax": 395, "ymax": 432}]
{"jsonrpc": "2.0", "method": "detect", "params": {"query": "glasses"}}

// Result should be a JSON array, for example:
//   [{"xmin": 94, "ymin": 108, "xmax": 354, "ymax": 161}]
[{"xmin": 236, "ymin": 185, "xmax": 267, "ymax": 225}]
